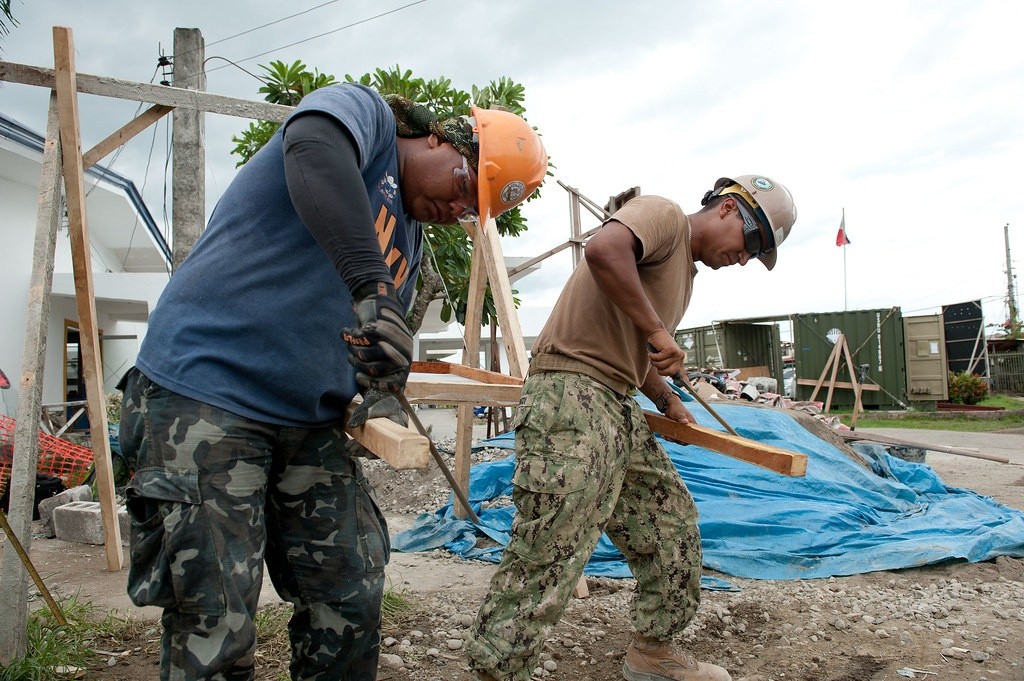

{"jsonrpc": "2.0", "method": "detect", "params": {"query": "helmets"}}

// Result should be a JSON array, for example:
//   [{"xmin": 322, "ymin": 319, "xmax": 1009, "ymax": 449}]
[
  {"xmin": 470, "ymin": 104, "xmax": 548, "ymax": 237},
  {"xmin": 714, "ymin": 174, "xmax": 797, "ymax": 271}
]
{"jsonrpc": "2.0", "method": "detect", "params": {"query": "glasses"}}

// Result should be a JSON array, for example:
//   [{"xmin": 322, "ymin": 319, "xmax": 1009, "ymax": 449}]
[
  {"xmin": 454, "ymin": 155, "xmax": 480, "ymax": 222},
  {"xmin": 728, "ymin": 193, "xmax": 764, "ymax": 259}
]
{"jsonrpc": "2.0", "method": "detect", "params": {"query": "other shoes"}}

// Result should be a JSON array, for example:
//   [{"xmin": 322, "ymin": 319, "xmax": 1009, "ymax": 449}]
[{"xmin": 622, "ymin": 631, "xmax": 733, "ymax": 681}]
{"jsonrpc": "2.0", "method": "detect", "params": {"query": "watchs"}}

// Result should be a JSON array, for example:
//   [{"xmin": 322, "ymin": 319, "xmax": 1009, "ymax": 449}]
[{"xmin": 655, "ymin": 390, "xmax": 681, "ymax": 413}]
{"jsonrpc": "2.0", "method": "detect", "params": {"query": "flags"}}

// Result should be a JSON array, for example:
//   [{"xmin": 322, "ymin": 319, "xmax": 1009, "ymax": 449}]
[{"xmin": 836, "ymin": 217, "xmax": 851, "ymax": 246}]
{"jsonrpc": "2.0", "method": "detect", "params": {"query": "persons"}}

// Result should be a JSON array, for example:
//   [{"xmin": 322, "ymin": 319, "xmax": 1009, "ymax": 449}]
[
  {"xmin": 466, "ymin": 175, "xmax": 797, "ymax": 681},
  {"xmin": 115, "ymin": 83, "xmax": 547, "ymax": 681}
]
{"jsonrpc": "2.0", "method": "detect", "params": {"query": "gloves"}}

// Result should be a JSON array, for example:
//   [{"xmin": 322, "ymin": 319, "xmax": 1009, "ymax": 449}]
[
  {"xmin": 343, "ymin": 387, "xmax": 410, "ymax": 460},
  {"xmin": 340, "ymin": 278, "xmax": 414, "ymax": 393}
]
